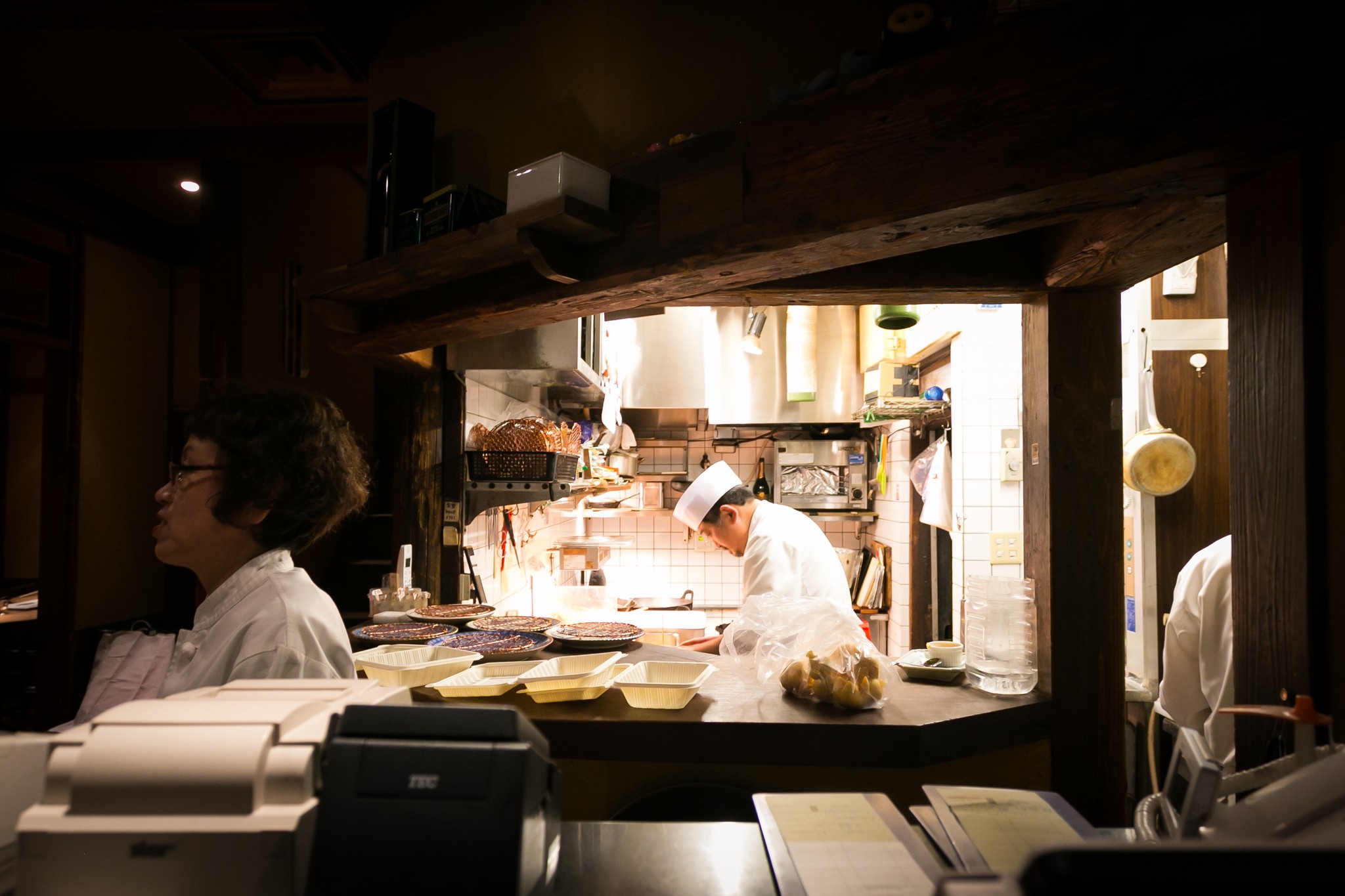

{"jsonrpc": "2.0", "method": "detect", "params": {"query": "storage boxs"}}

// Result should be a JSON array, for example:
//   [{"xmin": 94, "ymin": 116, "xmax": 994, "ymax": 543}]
[{"xmin": 465, "ymin": 451, "xmax": 580, "ymax": 482}]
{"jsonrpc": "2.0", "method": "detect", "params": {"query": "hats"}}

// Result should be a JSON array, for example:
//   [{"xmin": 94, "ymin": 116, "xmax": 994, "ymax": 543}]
[{"xmin": 671, "ymin": 460, "xmax": 743, "ymax": 531}]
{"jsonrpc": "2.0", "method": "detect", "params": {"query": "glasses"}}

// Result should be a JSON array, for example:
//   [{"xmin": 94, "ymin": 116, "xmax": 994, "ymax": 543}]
[{"xmin": 167, "ymin": 461, "xmax": 228, "ymax": 487}]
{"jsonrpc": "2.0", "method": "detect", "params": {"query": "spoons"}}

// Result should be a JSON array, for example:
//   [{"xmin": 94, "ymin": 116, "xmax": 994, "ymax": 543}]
[{"xmin": 891, "ymin": 658, "xmax": 942, "ymax": 668}]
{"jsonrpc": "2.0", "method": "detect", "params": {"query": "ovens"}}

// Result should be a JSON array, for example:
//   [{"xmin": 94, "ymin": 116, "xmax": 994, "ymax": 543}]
[{"xmin": 772, "ymin": 439, "xmax": 869, "ymax": 510}]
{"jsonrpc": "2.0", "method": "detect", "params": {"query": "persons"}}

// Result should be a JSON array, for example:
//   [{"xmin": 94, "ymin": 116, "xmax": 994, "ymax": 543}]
[
  {"xmin": 151, "ymin": 373, "xmax": 372, "ymax": 699},
  {"xmin": 1155, "ymin": 532, "xmax": 1236, "ymax": 781},
  {"xmin": 669, "ymin": 460, "xmax": 866, "ymax": 656}
]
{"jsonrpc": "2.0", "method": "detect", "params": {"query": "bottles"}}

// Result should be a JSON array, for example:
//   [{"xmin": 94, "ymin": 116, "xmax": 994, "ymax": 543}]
[
  {"xmin": 753, "ymin": 457, "xmax": 769, "ymax": 502},
  {"xmin": 962, "ymin": 573, "xmax": 1039, "ymax": 697}
]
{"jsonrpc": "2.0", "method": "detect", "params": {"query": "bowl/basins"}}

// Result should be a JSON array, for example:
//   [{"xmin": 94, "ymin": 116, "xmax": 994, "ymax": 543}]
[{"xmin": 926, "ymin": 639, "xmax": 964, "ymax": 668}]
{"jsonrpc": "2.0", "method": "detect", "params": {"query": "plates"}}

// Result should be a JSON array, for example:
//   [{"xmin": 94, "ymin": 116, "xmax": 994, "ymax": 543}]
[
  {"xmin": 350, "ymin": 602, "xmax": 646, "ymax": 661},
  {"xmin": 896, "ymin": 648, "xmax": 968, "ymax": 682}
]
